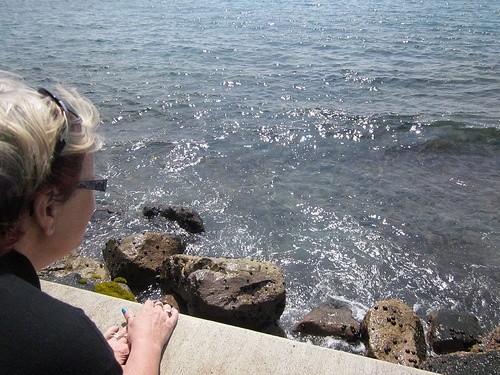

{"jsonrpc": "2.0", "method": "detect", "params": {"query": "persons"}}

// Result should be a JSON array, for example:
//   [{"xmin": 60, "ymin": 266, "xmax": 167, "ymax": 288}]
[{"xmin": 0, "ymin": 69, "xmax": 179, "ymax": 375}]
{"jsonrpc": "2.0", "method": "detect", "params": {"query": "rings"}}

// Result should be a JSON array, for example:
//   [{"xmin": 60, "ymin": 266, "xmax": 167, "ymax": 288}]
[
  {"xmin": 153, "ymin": 303, "xmax": 163, "ymax": 309},
  {"xmin": 165, "ymin": 309, "xmax": 172, "ymax": 317},
  {"xmin": 109, "ymin": 332, "xmax": 120, "ymax": 339},
  {"xmin": 120, "ymin": 334, "xmax": 129, "ymax": 341},
  {"xmin": 154, "ymin": 300, "xmax": 162, "ymax": 303},
  {"xmin": 126, "ymin": 315, "xmax": 136, "ymax": 323}
]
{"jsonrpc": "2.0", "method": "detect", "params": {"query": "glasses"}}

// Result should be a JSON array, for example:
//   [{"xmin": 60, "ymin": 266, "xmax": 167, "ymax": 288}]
[
  {"xmin": 36, "ymin": 87, "xmax": 83, "ymax": 184},
  {"xmin": 69, "ymin": 175, "xmax": 107, "ymax": 194}
]
